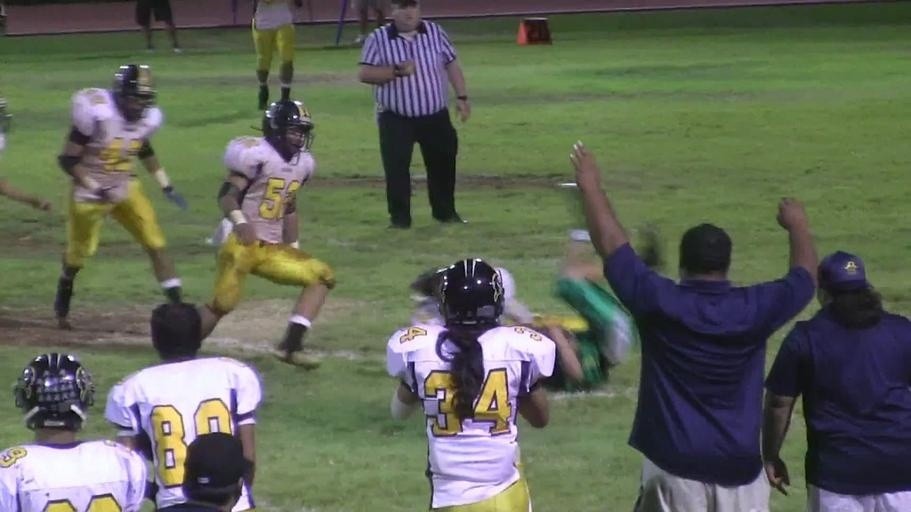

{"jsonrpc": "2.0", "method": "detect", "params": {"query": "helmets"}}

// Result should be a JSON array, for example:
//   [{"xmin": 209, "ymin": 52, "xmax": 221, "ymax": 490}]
[
  {"xmin": 264, "ymin": 101, "xmax": 311, "ymax": 158},
  {"xmin": 441, "ymin": 258, "xmax": 504, "ymax": 324},
  {"xmin": 113, "ymin": 64, "xmax": 158, "ymax": 121},
  {"xmin": 15, "ymin": 352, "xmax": 95, "ymax": 429}
]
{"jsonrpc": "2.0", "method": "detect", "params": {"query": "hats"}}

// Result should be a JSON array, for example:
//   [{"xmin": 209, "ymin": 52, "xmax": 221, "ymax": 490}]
[
  {"xmin": 183, "ymin": 432, "xmax": 254, "ymax": 481},
  {"xmin": 817, "ymin": 251, "xmax": 874, "ymax": 294}
]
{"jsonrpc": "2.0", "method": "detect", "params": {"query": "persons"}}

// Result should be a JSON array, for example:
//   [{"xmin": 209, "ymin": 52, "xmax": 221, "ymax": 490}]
[
  {"xmin": 566, "ymin": 137, "xmax": 818, "ymax": 509},
  {"xmin": 134, "ymin": 2, "xmax": 187, "ymax": 54},
  {"xmin": 106, "ymin": 301, "xmax": 264, "ymax": 510},
  {"xmin": 1, "ymin": 103, "xmax": 48, "ymax": 223},
  {"xmin": 0, "ymin": 351, "xmax": 148, "ymax": 512},
  {"xmin": 765, "ymin": 249, "xmax": 911, "ymax": 512},
  {"xmin": 247, "ymin": 2, "xmax": 299, "ymax": 110},
  {"xmin": 501, "ymin": 233, "xmax": 661, "ymax": 390},
  {"xmin": 194, "ymin": 98, "xmax": 335, "ymax": 371},
  {"xmin": 356, "ymin": 3, "xmax": 385, "ymax": 47},
  {"xmin": 385, "ymin": 256, "xmax": 557, "ymax": 511},
  {"xmin": 354, "ymin": 1, "xmax": 471, "ymax": 229},
  {"xmin": 158, "ymin": 431, "xmax": 252, "ymax": 511},
  {"xmin": 52, "ymin": 62, "xmax": 189, "ymax": 326}
]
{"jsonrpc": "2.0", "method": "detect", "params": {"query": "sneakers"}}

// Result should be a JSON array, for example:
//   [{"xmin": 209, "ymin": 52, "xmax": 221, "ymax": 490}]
[
  {"xmin": 275, "ymin": 345, "xmax": 322, "ymax": 371},
  {"xmin": 56, "ymin": 314, "xmax": 71, "ymax": 332}
]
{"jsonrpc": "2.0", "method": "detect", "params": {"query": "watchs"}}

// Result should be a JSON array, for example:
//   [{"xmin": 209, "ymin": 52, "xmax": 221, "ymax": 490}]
[{"xmin": 456, "ymin": 94, "xmax": 468, "ymax": 100}]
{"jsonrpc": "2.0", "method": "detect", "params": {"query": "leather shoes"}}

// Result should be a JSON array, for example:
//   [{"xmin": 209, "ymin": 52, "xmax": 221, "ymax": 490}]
[
  {"xmin": 387, "ymin": 219, "xmax": 412, "ymax": 230},
  {"xmin": 436, "ymin": 213, "xmax": 467, "ymax": 226}
]
